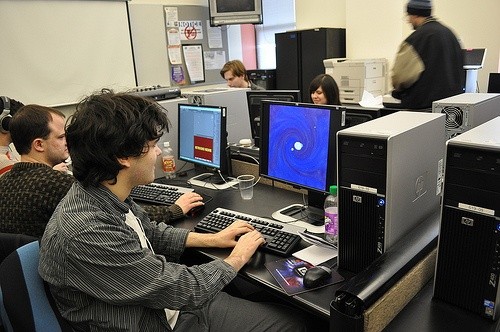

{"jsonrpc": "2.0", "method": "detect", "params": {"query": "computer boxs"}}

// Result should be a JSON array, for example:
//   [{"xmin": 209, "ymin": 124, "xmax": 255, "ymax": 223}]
[
  {"xmin": 432, "ymin": 116, "xmax": 500, "ymax": 324},
  {"xmin": 433, "ymin": 92, "xmax": 500, "ymax": 141},
  {"xmin": 336, "ymin": 111, "xmax": 446, "ymax": 276},
  {"xmin": 180, "ymin": 87, "xmax": 253, "ymax": 146},
  {"xmin": 153, "ymin": 97, "xmax": 188, "ymax": 181}
]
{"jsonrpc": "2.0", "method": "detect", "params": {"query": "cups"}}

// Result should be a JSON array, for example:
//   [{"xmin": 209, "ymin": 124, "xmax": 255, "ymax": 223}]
[{"xmin": 237, "ymin": 174, "xmax": 255, "ymax": 201}]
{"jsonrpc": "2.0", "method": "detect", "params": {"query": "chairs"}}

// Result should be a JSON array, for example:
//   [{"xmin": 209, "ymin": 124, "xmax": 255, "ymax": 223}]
[{"xmin": 0, "ymin": 240, "xmax": 74, "ymax": 332}]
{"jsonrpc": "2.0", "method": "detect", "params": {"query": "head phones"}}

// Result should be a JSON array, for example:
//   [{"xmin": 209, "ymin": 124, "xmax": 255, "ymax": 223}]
[{"xmin": 0, "ymin": 96, "xmax": 13, "ymax": 134}]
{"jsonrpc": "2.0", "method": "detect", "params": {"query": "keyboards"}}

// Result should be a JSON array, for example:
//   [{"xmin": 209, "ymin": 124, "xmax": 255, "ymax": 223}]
[
  {"xmin": 129, "ymin": 182, "xmax": 195, "ymax": 206},
  {"xmin": 194, "ymin": 207, "xmax": 307, "ymax": 257}
]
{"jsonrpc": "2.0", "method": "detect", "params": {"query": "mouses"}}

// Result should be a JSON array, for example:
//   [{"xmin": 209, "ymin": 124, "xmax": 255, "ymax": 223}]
[
  {"xmin": 303, "ymin": 266, "xmax": 332, "ymax": 289},
  {"xmin": 186, "ymin": 205, "xmax": 205, "ymax": 217}
]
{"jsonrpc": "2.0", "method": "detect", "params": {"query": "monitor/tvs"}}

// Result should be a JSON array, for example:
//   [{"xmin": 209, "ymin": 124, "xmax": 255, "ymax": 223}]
[
  {"xmin": 208, "ymin": 0, "xmax": 263, "ymax": 27},
  {"xmin": 178, "ymin": 103, "xmax": 239, "ymax": 190},
  {"xmin": 462, "ymin": 48, "xmax": 487, "ymax": 70},
  {"xmin": 246, "ymin": 89, "xmax": 381, "ymax": 233}
]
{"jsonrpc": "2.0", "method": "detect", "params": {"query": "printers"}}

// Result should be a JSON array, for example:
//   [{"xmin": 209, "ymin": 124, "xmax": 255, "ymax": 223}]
[{"xmin": 323, "ymin": 57, "xmax": 389, "ymax": 104}]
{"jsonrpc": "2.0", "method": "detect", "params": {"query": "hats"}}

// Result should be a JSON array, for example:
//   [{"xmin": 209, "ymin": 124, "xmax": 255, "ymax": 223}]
[{"xmin": 407, "ymin": 0, "xmax": 431, "ymax": 16}]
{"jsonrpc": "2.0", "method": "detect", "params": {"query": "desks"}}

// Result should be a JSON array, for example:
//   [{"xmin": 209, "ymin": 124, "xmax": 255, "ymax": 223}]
[{"xmin": 132, "ymin": 166, "xmax": 343, "ymax": 317}]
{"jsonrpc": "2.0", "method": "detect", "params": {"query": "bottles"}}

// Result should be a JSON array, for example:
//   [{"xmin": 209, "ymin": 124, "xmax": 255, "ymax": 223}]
[
  {"xmin": 324, "ymin": 185, "xmax": 338, "ymax": 245},
  {"xmin": 160, "ymin": 142, "xmax": 176, "ymax": 179}
]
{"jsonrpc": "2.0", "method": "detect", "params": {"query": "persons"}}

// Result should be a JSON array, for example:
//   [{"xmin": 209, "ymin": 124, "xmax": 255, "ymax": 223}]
[
  {"xmin": 38, "ymin": 88, "xmax": 310, "ymax": 332},
  {"xmin": 221, "ymin": 61, "xmax": 265, "ymax": 90},
  {"xmin": 0, "ymin": 95, "xmax": 69, "ymax": 178},
  {"xmin": 391, "ymin": 0, "xmax": 463, "ymax": 113},
  {"xmin": 308, "ymin": 74, "xmax": 343, "ymax": 107},
  {"xmin": 0, "ymin": 104, "xmax": 205, "ymax": 237}
]
